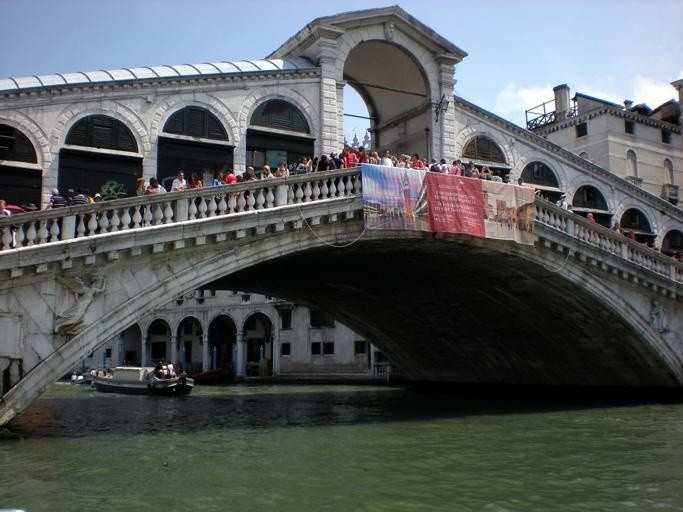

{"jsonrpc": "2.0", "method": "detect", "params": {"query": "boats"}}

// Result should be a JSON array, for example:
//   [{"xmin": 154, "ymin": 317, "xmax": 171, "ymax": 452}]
[
  {"xmin": 71, "ymin": 372, "xmax": 90, "ymax": 386},
  {"xmin": 91, "ymin": 365, "xmax": 194, "ymax": 394}
]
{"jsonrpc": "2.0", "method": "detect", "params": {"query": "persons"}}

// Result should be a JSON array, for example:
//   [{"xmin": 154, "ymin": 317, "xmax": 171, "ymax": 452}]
[
  {"xmin": 586, "ymin": 213, "xmax": 596, "ymax": 222},
  {"xmin": 44, "ymin": 188, "xmax": 103, "ymax": 210},
  {"xmin": 557, "ymin": 191, "xmax": 573, "ymax": 212},
  {"xmin": 613, "ymin": 222, "xmax": 636, "ymax": 240}
]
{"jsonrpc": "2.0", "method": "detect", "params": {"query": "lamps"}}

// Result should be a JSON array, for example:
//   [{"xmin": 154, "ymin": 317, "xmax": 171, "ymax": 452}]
[{"xmin": 436, "ymin": 94, "xmax": 449, "ymax": 123}]
